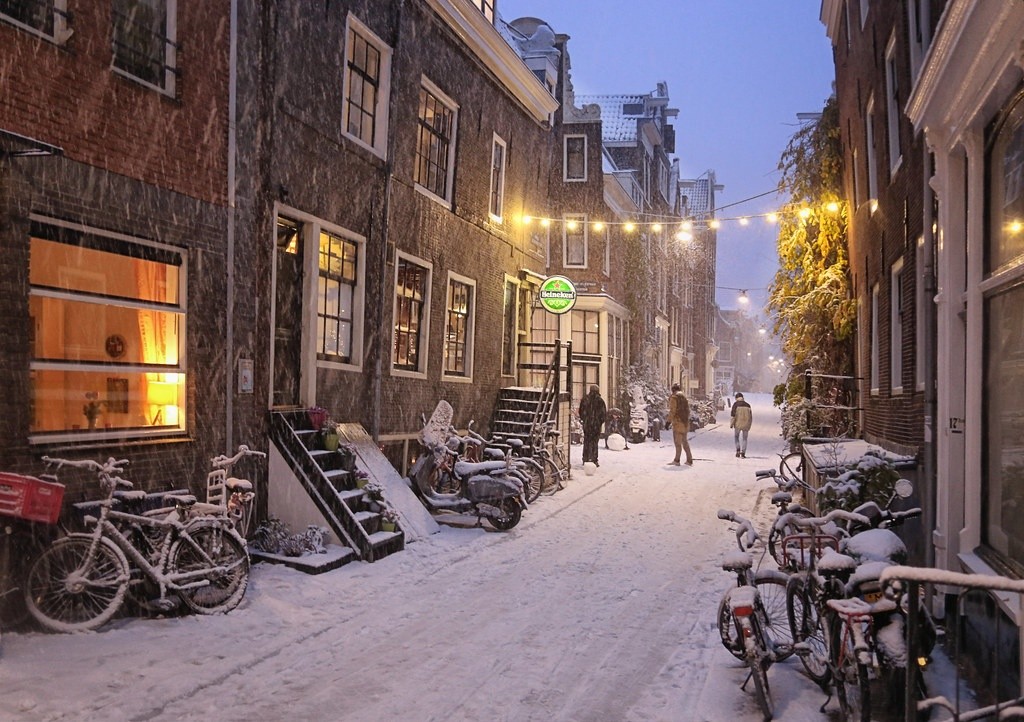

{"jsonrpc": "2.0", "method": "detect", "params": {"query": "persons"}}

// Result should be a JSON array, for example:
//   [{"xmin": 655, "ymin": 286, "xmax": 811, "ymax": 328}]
[
  {"xmin": 605, "ymin": 408, "xmax": 630, "ymax": 449},
  {"xmin": 664, "ymin": 383, "xmax": 693, "ymax": 466},
  {"xmin": 730, "ymin": 393, "xmax": 753, "ymax": 458},
  {"xmin": 578, "ymin": 384, "xmax": 607, "ymax": 468}
]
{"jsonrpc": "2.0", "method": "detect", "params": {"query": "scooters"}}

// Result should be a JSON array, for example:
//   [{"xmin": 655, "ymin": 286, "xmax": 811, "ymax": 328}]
[
  {"xmin": 402, "ymin": 400, "xmax": 528, "ymax": 531},
  {"xmin": 628, "ymin": 401, "xmax": 653, "ymax": 444}
]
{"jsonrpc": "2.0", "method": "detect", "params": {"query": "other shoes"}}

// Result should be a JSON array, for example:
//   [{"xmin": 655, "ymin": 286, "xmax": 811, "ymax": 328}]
[
  {"xmin": 591, "ymin": 460, "xmax": 599, "ymax": 467},
  {"xmin": 736, "ymin": 451, "xmax": 740, "ymax": 457},
  {"xmin": 740, "ymin": 452, "xmax": 746, "ymax": 458},
  {"xmin": 583, "ymin": 459, "xmax": 588, "ymax": 465},
  {"xmin": 667, "ymin": 460, "xmax": 681, "ymax": 467},
  {"xmin": 684, "ymin": 461, "xmax": 692, "ymax": 466}
]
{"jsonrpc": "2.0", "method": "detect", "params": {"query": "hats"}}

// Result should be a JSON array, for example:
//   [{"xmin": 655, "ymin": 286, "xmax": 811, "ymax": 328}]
[
  {"xmin": 590, "ymin": 383, "xmax": 599, "ymax": 391},
  {"xmin": 735, "ymin": 393, "xmax": 743, "ymax": 398},
  {"xmin": 671, "ymin": 382, "xmax": 680, "ymax": 389}
]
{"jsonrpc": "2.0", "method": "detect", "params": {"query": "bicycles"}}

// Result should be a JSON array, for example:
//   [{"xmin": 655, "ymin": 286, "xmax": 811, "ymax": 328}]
[
  {"xmin": 697, "ymin": 399, "xmax": 718, "ymax": 426},
  {"xmin": 771, "ymin": 420, "xmax": 859, "ymax": 481},
  {"xmin": 433, "ymin": 420, "xmax": 570, "ymax": 503},
  {"xmin": 22, "ymin": 442, "xmax": 268, "ymax": 633},
  {"xmin": 717, "ymin": 451, "xmax": 938, "ymax": 721}
]
